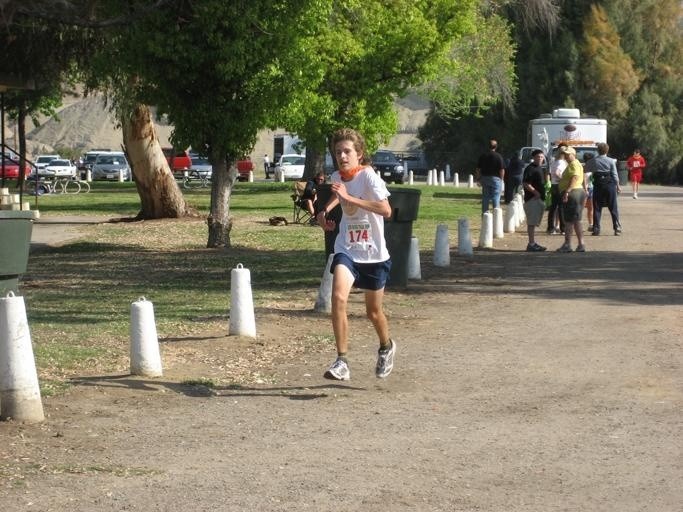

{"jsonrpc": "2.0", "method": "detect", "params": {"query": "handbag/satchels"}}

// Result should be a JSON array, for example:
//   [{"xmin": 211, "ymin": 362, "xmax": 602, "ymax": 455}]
[{"xmin": 269, "ymin": 216, "xmax": 288, "ymax": 226}]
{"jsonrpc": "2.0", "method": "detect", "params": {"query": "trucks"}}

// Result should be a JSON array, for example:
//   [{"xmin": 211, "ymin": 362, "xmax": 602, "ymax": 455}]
[{"xmin": 519, "ymin": 109, "xmax": 608, "ymax": 182}]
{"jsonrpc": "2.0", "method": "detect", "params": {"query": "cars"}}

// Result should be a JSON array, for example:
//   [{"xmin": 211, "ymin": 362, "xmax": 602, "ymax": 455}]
[
  {"xmin": 90, "ymin": 153, "xmax": 130, "ymax": 181},
  {"xmin": 368, "ymin": 149, "xmax": 403, "ymax": 184},
  {"xmin": 273, "ymin": 154, "xmax": 306, "ymax": 180},
  {"xmin": 45, "ymin": 159, "xmax": 77, "ymax": 180},
  {"xmin": 188, "ymin": 147, "xmax": 208, "ymax": 158},
  {"xmin": 190, "ymin": 157, "xmax": 212, "ymax": 179},
  {"xmin": 160, "ymin": 147, "xmax": 191, "ymax": 178},
  {"xmin": 398, "ymin": 156, "xmax": 421, "ymax": 174},
  {"xmin": 0, "ymin": 158, "xmax": 32, "ymax": 181},
  {"xmin": 237, "ymin": 156, "xmax": 254, "ymax": 180}
]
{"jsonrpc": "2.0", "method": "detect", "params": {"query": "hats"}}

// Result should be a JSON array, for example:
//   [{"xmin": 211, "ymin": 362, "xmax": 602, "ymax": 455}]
[{"xmin": 564, "ymin": 147, "xmax": 576, "ymax": 155}]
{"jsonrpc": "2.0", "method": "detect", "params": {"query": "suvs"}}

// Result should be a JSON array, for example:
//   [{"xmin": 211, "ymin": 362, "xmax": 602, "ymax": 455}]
[
  {"xmin": 79, "ymin": 151, "xmax": 108, "ymax": 180},
  {"xmin": 33, "ymin": 155, "xmax": 62, "ymax": 176}
]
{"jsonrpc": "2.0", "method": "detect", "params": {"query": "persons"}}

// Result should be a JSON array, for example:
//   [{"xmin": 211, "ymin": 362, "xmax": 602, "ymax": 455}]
[
  {"xmin": 474, "ymin": 139, "xmax": 622, "ymax": 253},
  {"xmin": 300, "ymin": 173, "xmax": 326, "ymax": 227},
  {"xmin": 382, "ymin": 154, "xmax": 391, "ymax": 163},
  {"xmin": 263, "ymin": 154, "xmax": 270, "ymax": 180},
  {"xmin": 67, "ymin": 155, "xmax": 94, "ymax": 181},
  {"xmin": 315, "ymin": 128, "xmax": 396, "ymax": 381},
  {"xmin": 624, "ymin": 147, "xmax": 646, "ymax": 202}
]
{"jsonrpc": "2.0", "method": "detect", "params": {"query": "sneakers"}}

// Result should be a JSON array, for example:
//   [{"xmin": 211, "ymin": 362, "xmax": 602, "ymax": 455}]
[
  {"xmin": 615, "ymin": 231, "xmax": 621, "ymax": 235},
  {"xmin": 527, "ymin": 243, "xmax": 547, "ymax": 251},
  {"xmin": 557, "ymin": 243, "xmax": 572, "ymax": 252},
  {"xmin": 587, "ymin": 225, "xmax": 600, "ymax": 235},
  {"xmin": 546, "ymin": 226, "xmax": 565, "ymax": 234},
  {"xmin": 324, "ymin": 360, "xmax": 350, "ymax": 380},
  {"xmin": 575, "ymin": 244, "xmax": 585, "ymax": 252},
  {"xmin": 376, "ymin": 338, "xmax": 396, "ymax": 378}
]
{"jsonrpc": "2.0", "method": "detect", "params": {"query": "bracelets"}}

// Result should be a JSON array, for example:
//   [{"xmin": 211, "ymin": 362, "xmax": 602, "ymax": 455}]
[{"xmin": 320, "ymin": 208, "xmax": 330, "ymax": 214}]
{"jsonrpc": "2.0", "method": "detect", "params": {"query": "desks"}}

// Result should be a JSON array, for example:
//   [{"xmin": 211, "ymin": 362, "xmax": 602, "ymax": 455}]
[{"xmin": 179, "ymin": 170, "xmax": 209, "ymax": 188}]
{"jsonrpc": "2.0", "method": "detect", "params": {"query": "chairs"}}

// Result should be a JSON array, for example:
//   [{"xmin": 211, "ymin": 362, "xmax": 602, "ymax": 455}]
[{"xmin": 291, "ymin": 182, "xmax": 314, "ymax": 224}]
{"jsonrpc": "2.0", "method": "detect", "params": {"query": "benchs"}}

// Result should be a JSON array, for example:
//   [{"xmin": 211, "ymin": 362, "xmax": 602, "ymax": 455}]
[{"xmin": 175, "ymin": 175, "xmax": 207, "ymax": 179}]
